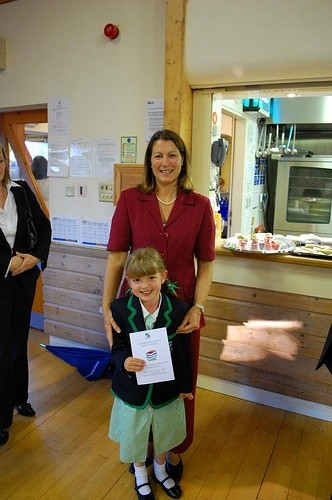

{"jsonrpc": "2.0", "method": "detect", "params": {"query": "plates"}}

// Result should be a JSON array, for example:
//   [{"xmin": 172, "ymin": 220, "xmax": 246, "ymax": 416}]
[{"xmin": 222, "ymin": 233, "xmax": 296, "ymax": 255}]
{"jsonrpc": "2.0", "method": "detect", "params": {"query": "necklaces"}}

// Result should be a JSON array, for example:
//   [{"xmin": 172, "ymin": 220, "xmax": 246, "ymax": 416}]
[{"xmin": 155, "ymin": 192, "xmax": 177, "ymax": 205}]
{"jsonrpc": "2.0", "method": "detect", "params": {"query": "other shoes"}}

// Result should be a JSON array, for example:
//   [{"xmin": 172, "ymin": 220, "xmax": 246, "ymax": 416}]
[
  {"xmin": 129, "ymin": 451, "xmax": 153, "ymax": 473},
  {"xmin": 0, "ymin": 427, "xmax": 10, "ymax": 446},
  {"xmin": 135, "ymin": 476, "xmax": 155, "ymax": 500},
  {"xmin": 166, "ymin": 455, "xmax": 184, "ymax": 481},
  {"xmin": 153, "ymin": 472, "xmax": 182, "ymax": 500},
  {"xmin": 14, "ymin": 401, "xmax": 37, "ymax": 417}
]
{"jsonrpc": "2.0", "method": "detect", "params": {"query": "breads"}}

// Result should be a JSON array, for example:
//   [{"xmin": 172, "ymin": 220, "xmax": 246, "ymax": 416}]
[{"xmin": 305, "ymin": 244, "xmax": 332, "ymax": 256}]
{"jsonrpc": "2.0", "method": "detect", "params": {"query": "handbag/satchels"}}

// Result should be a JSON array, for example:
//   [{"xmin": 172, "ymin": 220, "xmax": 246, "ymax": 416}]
[{"xmin": 24, "ymin": 207, "xmax": 39, "ymax": 254}]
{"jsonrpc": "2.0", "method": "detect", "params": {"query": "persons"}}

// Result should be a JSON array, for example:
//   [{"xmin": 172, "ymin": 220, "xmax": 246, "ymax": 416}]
[
  {"xmin": 108, "ymin": 248, "xmax": 194, "ymax": 500},
  {"xmin": 102, "ymin": 129, "xmax": 215, "ymax": 482},
  {"xmin": 0, "ymin": 143, "xmax": 52, "ymax": 447}
]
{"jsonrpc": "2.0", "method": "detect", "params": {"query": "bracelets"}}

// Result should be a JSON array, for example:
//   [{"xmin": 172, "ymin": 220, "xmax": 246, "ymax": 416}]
[{"xmin": 194, "ymin": 302, "xmax": 205, "ymax": 312}]
